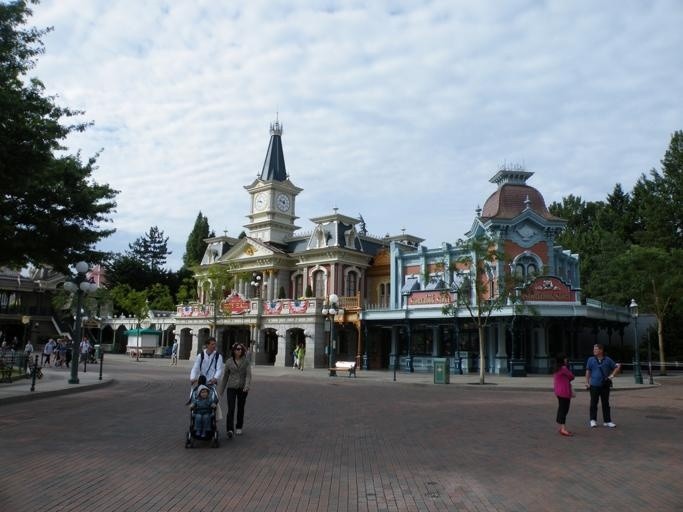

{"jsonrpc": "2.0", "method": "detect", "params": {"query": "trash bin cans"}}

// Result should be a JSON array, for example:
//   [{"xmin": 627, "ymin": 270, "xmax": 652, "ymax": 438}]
[
  {"xmin": 433, "ymin": 358, "xmax": 450, "ymax": 384},
  {"xmin": 94, "ymin": 344, "xmax": 100, "ymax": 359}
]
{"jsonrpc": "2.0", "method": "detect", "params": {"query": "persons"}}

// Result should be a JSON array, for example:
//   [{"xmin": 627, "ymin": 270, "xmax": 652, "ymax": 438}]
[
  {"xmin": 0, "ymin": 335, "xmax": 97, "ymax": 368},
  {"xmin": 216, "ymin": 342, "xmax": 251, "ymax": 439},
  {"xmin": 189, "ymin": 384, "xmax": 217, "ymax": 439},
  {"xmin": 292, "ymin": 345, "xmax": 299, "ymax": 370},
  {"xmin": 553, "ymin": 352, "xmax": 575, "ymax": 436},
  {"xmin": 294, "ymin": 343, "xmax": 305, "ymax": 371},
  {"xmin": 584, "ymin": 343, "xmax": 620, "ymax": 429},
  {"xmin": 169, "ymin": 339, "xmax": 177, "ymax": 367},
  {"xmin": 188, "ymin": 337, "xmax": 223, "ymax": 386}
]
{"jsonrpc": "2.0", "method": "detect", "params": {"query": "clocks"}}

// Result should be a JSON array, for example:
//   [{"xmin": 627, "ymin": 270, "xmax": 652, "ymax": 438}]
[
  {"xmin": 254, "ymin": 193, "xmax": 268, "ymax": 211},
  {"xmin": 275, "ymin": 194, "xmax": 290, "ymax": 212}
]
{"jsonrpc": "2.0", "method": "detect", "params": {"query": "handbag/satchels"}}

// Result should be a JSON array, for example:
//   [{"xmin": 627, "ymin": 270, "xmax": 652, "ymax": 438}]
[{"xmin": 602, "ymin": 376, "xmax": 613, "ymax": 387}]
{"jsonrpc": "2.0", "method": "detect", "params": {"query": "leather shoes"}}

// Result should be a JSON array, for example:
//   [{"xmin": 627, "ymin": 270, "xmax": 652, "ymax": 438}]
[{"xmin": 559, "ymin": 429, "xmax": 572, "ymax": 435}]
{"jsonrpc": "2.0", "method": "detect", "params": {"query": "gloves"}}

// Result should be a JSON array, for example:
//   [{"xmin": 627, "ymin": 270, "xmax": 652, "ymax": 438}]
[
  {"xmin": 191, "ymin": 403, "xmax": 195, "ymax": 408},
  {"xmin": 211, "ymin": 403, "xmax": 216, "ymax": 408}
]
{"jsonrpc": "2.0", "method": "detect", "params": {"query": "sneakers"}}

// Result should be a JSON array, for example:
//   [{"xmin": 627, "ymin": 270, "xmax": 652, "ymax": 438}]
[
  {"xmin": 591, "ymin": 420, "xmax": 596, "ymax": 427},
  {"xmin": 235, "ymin": 429, "xmax": 242, "ymax": 435},
  {"xmin": 196, "ymin": 430, "xmax": 206, "ymax": 437},
  {"xmin": 603, "ymin": 422, "xmax": 615, "ymax": 427},
  {"xmin": 227, "ymin": 431, "xmax": 233, "ymax": 438}
]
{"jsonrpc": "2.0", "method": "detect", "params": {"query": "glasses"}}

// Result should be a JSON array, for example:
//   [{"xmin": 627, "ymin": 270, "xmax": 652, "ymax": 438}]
[{"xmin": 236, "ymin": 348, "xmax": 243, "ymax": 351}]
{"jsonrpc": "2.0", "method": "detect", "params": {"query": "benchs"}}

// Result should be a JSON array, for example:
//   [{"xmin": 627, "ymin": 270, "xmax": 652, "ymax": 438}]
[
  {"xmin": 328, "ymin": 361, "xmax": 356, "ymax": 378},
  {"xmin": 29, "ymin": 356, "xmax": 43, "ymax": 379}
]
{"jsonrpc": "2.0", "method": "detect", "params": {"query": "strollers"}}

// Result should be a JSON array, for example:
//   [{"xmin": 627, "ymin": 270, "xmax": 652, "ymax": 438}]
[{"xmin": 185, "ymin": 384, "xmax": 219, "ymax": 449}]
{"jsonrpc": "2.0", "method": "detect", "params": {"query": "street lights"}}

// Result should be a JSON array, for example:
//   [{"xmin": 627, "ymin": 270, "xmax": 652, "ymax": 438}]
[
  {"xmin": 629, "ymin": 298, "xmax": 643, "ymax": 384},
  {"xmin": 63, "ymin": 261, "xmax": 97, "ymax": 384},
  {"xmin": 321, "ymin": 294, "xmax": 344, "ymax": 377}
]
